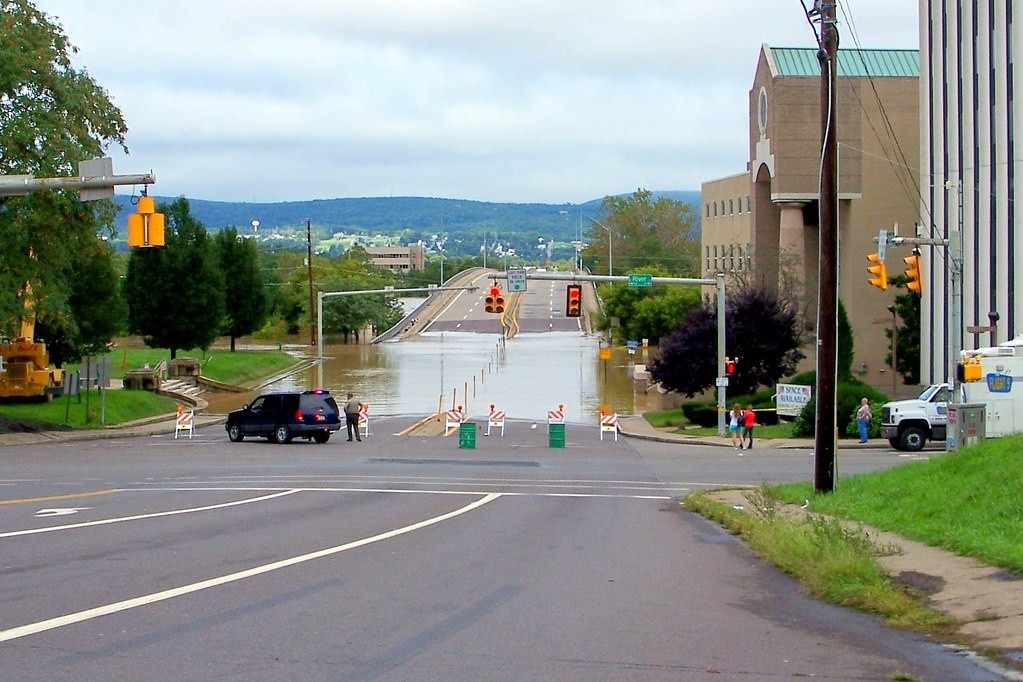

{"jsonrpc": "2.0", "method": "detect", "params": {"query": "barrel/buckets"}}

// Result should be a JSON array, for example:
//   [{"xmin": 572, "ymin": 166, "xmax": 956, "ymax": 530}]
[
  {"xmin": 548, "ymin": 424, "xmax": 565, "ymax": 448},
  {"xmin": 459, "ymin": 423, "xmax": 476, "ymax": 449}
]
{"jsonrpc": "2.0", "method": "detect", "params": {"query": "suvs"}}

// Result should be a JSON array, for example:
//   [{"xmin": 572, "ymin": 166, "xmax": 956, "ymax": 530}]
[
  {"xmin": 224, "ymin": 389, "xmax": 342, "ymax": 444},
  {"xmin": 878, "ymin": 382, "xmax": 969, "ymax": 452}
]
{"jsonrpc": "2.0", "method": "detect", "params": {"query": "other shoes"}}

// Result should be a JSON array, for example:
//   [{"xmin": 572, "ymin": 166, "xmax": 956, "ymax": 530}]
[
  {"xmin": 859, "ymin": 441, "xmax": 865, "ymax": 443},
  {"xmin": 357, "ymin": 438, "xmax": 361, "ymax": 441},
  {"xmin": 742, "ymin": 447, "xmax": 747, "ymax": 449},
  {"xmin": 347, "ymin": 439, "xmax": 352, "ymax": 441}
]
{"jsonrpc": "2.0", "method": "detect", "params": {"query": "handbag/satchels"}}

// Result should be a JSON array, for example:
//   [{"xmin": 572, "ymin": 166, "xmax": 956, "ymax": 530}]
[{"xmin": 737, "ymin": 412, "xmax": 744, "ymax": 425}]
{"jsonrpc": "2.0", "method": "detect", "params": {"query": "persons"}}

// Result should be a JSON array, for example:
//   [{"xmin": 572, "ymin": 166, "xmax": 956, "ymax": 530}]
[
  {"xmin": 730, "ymin": 403, "xmax": 748, "ymax": 450},
  {"xmin": 738, "ymin": 404, "xmax": 756, "ymax": 450},
  {"xmin": 857, "ymin": 397, "xmax": 871, "ymax": 443},
  {"xmin": 343, "ymin": 392, "xmax": 363, "ymax": 442}
]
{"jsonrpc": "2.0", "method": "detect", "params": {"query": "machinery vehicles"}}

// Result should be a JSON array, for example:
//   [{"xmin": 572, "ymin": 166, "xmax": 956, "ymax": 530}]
[{"xmin": 0, "ymin": 243, "xmax": 67, "ymax": 403}]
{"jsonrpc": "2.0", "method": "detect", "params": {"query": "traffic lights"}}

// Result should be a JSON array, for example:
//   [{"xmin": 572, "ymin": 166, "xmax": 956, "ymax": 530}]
[
  {"xmin": 484, "ymin": 285, "xmax": 506, "ymax": 314},
  {"xmin": 866, "ymin": 250, "xmax": 888, "ymax": 291},
  {"xmin": 903, "ymin": 253, "xmax": 925, "ymax": 298},
  {"xmin": 956, "ymin": 363, "xmax": 983, "ymax": 383},
  {"xmin": 566, "ymin": 284, "xmax": 582, "ymax": 318},
  {"xmin": 726, "ymin": 362, "xmax": 735, "ymax": 374}
]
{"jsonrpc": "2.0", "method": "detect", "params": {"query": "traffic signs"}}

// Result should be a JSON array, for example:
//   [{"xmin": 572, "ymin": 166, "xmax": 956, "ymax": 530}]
[{"xmin": 628, "ymin": 274, "xmax": 653, "ymax": 288}]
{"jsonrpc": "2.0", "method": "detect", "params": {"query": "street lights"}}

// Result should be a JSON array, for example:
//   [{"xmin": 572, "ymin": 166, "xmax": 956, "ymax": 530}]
[
  {"xmin": 483, "ymin": 207, "xmax": 498, "ymax": 269},
  {"xmin": 987, "ymin": 310, "xmax": 1001, "ymax": 347},
  {"xmin": 440, "ymin": 204, "xmax": 462, "ymax": 285},
  {"xmin": 565, "ymin": 218, "xmax": 577, "ymax": 269},
  {"xmin": 885, "ymin": 304, "xmax": 898, "ymax": 402}
]
